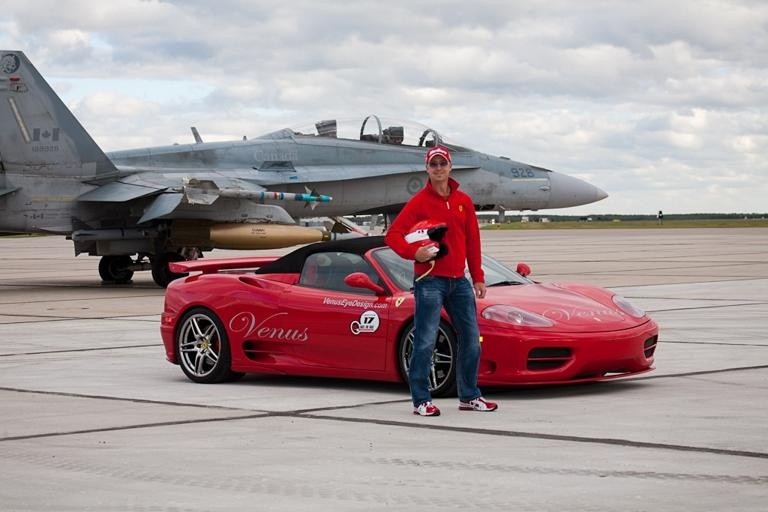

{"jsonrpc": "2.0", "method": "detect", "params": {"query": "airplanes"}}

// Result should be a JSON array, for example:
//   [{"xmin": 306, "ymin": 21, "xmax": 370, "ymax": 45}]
[{"xmin": 0, "ymin": 50, "xmax": 609, "ymax": 289}]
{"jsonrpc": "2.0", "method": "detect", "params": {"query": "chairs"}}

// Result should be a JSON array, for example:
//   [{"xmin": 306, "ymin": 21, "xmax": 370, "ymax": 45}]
[{"xmin": 322, "ymin": 254, "xmax": 359, "ymax": 294}]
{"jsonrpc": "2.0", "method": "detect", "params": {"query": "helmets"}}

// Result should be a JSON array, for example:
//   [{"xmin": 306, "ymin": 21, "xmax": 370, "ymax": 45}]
[{"xmin": 404, "ymin": 220, "xmax": 449, "ymax": 264}]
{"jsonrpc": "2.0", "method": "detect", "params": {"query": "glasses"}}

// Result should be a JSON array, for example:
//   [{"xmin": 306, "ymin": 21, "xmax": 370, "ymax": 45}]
[{"xmin": 429, "ymin": 162, "xmax": 449, "ymax": 167}]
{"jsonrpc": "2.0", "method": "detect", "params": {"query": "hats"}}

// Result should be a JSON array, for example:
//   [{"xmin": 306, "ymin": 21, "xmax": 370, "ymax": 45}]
[{"xmin": 425, "ymin": 145, "xmax": 452, "ymax": 166}]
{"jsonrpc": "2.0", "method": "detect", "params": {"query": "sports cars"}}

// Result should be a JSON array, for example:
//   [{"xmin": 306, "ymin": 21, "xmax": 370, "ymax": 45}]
[{"xmin": 160, "ymin": 233, "xmax": 660, "ymax": 397}]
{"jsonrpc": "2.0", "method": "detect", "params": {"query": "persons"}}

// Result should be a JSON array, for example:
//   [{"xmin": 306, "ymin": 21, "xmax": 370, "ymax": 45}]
[{"xmin": 384, "ymin": 145, "xmax": 497, "ymax": 416}]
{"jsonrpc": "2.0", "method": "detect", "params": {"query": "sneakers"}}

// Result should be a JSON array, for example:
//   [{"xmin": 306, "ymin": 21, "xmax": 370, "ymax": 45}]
[
  {"xmin": 413, "ymin": 400, "xmax": 440, "ymax": 416},
  {"xmin": 459, "ymin": 396, "xmax": 498, "ymax": 412}
]
